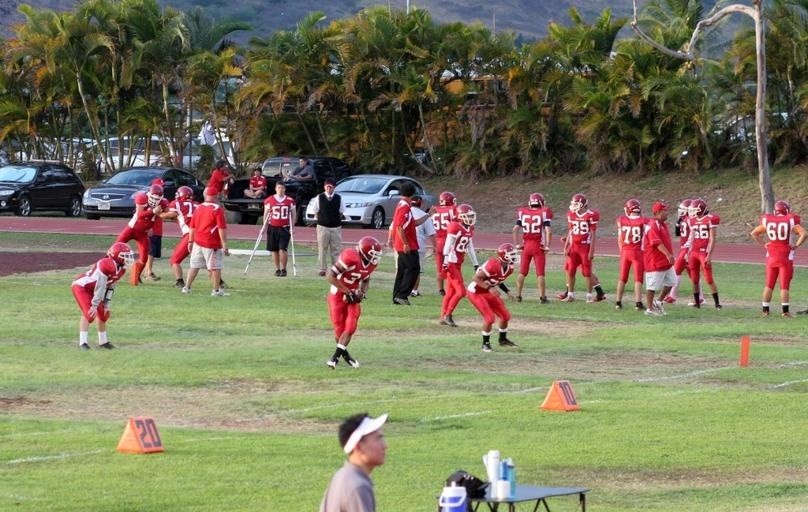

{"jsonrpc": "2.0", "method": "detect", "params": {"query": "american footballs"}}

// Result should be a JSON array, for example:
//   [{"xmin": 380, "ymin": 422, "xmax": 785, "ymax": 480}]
[{"xmin": 350, "ymin": 288, "xmax": 364, "ymax": 301}]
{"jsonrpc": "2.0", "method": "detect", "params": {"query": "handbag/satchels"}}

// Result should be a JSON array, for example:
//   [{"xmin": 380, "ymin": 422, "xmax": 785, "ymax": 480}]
[{"xmin": 448, "ymin": 472, "xmax": 488, "ymax": 499}]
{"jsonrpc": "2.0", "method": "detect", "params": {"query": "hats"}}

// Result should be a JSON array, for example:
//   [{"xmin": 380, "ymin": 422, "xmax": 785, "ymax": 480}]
[
  {"xmin": 653, "ymin": 203, "xmax": 666, "ymax": 214},
  {"xmin": 343, "ymin": 413, "xmax": 389, "ymax": 455},
  {"xmin": 206, "ymin": 186, "xmax": 219, "ymax": 195}
]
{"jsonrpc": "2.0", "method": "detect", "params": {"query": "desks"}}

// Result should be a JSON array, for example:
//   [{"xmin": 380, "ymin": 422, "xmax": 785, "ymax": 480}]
[{"xmin": 469, "ymin": 483, "xmax": 591, "ymax": 512}]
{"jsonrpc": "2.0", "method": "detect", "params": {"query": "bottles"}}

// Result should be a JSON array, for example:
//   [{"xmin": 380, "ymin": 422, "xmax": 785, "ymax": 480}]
[
  {"xmin": 483, "ymin": 450, "xmax": 515, "ymax": 499},
  {"xmin": 439, "ymin": 482, "xmax": 467, "ymax": 512}
]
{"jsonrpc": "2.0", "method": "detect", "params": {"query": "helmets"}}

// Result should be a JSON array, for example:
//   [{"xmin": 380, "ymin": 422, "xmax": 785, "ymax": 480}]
[
  {"xmin": 147, "ymin": 184, "xmax": 164, "ymax": 206},
  {"xmin": 107, "ymin": 243, "xmax": 133, "ymax": 269},
  {"xmin": 357, "ymin": 237, "xmax": 383, "ymax": 267},
  {"xmin": 174, "ymin": 186, "xmax": 192, "ymax": 202},
  {"xmin": 677, "ymin": 199, "xmax": 707, "ymax": 218},
  {"xmin": 439, "ymin": 192, "xmax": 456, "ymax": 205},
  {"xmin": 456, "ymin": 203, "xmax": 476, "ymax": 224},
  {"xmin": 568, "ymin": 193, "xmax": 589, "ymax": 214},
  {"xmin": 497, "ymin": 244, "xmax": 519, "ymax": 267},
  {"xmin": 529, "ymin": 192, "xmax": 543, "ymax": 209},
  {"xmin": 624, "ymin": 199, "xmax": 640, "ymax": 217},
  {"xmin": 774, "ymin": 201, "xmax": 790, "ymax": 215}
]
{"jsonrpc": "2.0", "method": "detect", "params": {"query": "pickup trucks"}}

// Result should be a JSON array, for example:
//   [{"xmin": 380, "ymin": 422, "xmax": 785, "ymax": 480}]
[{"xmin": 223, "ymin": 154, "xmax": 358, "ymax": 227}]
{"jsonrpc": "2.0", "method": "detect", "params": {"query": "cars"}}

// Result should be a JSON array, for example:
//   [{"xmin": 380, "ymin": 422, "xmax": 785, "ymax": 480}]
[
  {"xmin": 305, "ymin": 173, "xmax": 434, "ymax": 230},
  {"xmin": 711, "ymin": 111, "xmax": 789, "ymax": 144},
  {"xmin": 81, "ymin": 166, "xmax": 204, "ymax": 220},
  {"xmin": 11, "ymin": 130, "xmax": 238, "ymax": 173},
  {"xmin": 0, "ymin": 158, "xmax": 82, "ymax": 220}
]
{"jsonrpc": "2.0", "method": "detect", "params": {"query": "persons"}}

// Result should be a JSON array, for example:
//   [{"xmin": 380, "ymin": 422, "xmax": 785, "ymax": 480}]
[
  {"xmin": 105, "ymin": 155, "xmax": 345, "ymax": 296},
  {"xmin": 513, "ymin": 193, "xmax": 553, "ymax": 305},
  {"xmin": 388, "ymin": 183, "xmax": 436, "ymax": 305},
  {"xmin": 325, "ymin": 236, "xmax": 383, "ymax": 369},
  {"xmin": 427, "ymin": 191, "xmax": 480, "ymax": 326},
  {"xmin": 750, "ymin": 200, "xmax": 807, "ymax": 316},
  {"xmin": 557, "ymin": 194, "xmax": 606, "ymax": 303},
  {"xmin": 320, "ymin": 412, "xmax": 389, "ymax": 512},
  {"xmin": 467, "ymin": 244, "xmax": 518, "ymax": 351},
  {"xmin": 614, "ymin": 199, "xmax": 721, "ymax": 314},
  {"xmin": 70, "ymin": 242, "xmax": 134, "ymax": 350}
]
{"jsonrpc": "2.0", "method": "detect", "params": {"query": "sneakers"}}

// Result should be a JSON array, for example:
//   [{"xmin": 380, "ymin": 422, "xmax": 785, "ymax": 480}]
[
  {"xmin": 146, "ymin": 272, "xmax": 228, "ymax": 296},
  {"xmin": 98, "ymin": 343, "xmax": 114, "ymax": 349},
  {"xmin": 516, "ymin": 287, "xmax": 721, "ymax": 316},
  {"xmin": 275, "ymin": 269, "xmax": 285, "ymax": 275},
  {"xmin": 328, "ymin": 360, "xmax": 337, "ymax": 368},
  {"xmin": 482, "ymin": 343, "xmax": 492, "ymax": 352},
  {"xmin": 78, "ymin": 343, "xmax": 89, "ymax": 348},
  {"xmin": 343, "ymin": 354, "xmax": 358, "ymax": 368},
  {"xmin": 500, "ymin": 339, "xmax": 516, "ymax": 346},
  {"xmin": 762, "ymin": 311, "xmax": 795, "ymax": 318},
  {"xmin": 393, "ymin": 288, "xmax": 458, "ymax": 328}
]
